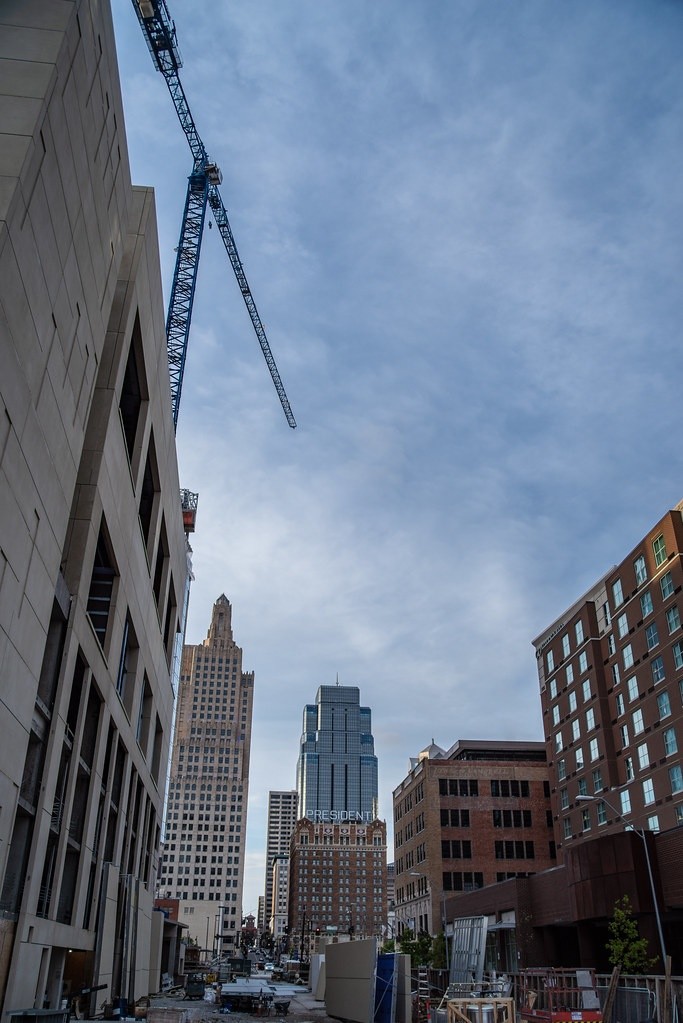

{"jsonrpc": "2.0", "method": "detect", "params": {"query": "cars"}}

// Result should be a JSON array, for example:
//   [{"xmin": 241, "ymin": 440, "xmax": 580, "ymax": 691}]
[
  {"xmin": 264, "ymin": 963, "xmax": 275, "ymax": 971},
  {"xmin": 250, "ymin": 948, "xmax": 278, "ymax": 970}
]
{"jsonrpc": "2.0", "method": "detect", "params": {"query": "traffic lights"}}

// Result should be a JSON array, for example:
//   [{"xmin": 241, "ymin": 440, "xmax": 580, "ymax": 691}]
[
  {"xmin": 316, "ymin": 928, "xmax": 320, "ymax": 936},
  {"xmin": 285, "ymin": 926, "xmax": 289, "ymax": 934},
  {"xmin": 351, "ymin": 926, "xmax": 354, "ymax": 933}
]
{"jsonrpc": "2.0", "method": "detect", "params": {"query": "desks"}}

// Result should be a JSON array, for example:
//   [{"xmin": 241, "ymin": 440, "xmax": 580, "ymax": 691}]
[{"xmin": 447, "ymin": 997, "xmax": 513, "ymax": 1023}]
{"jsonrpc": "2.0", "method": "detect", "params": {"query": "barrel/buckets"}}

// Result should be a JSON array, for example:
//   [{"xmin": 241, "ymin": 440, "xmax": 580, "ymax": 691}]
[{"xmin": 112, "ymin": 997, "xmax": 128, "ymax": 1021}]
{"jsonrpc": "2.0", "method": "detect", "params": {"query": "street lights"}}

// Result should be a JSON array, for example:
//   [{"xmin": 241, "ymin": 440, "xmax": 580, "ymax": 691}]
[
  {"xmin": 217, "ymin": 906, "xmax": 226, "ymax": 949},
  {"xmin": 410, "ymin": 873, "xmax": 449, "ymax": 971},
  {"xmin": 213, "ymin": 915, "xmax": 220, "ymax": 954},
  {"xmin": 205, "ymin": 917, "xmax": 209, "ymax": 958},
  {"xmin": 575, "ymin": 795, "xmax": 666, "ymax": 966}
]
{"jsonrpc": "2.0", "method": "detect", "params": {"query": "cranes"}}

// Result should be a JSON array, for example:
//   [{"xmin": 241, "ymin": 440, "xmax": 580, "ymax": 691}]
[{"xmin": 131, "ymin": 0, "xmax": 298, "ymax": 434}]
